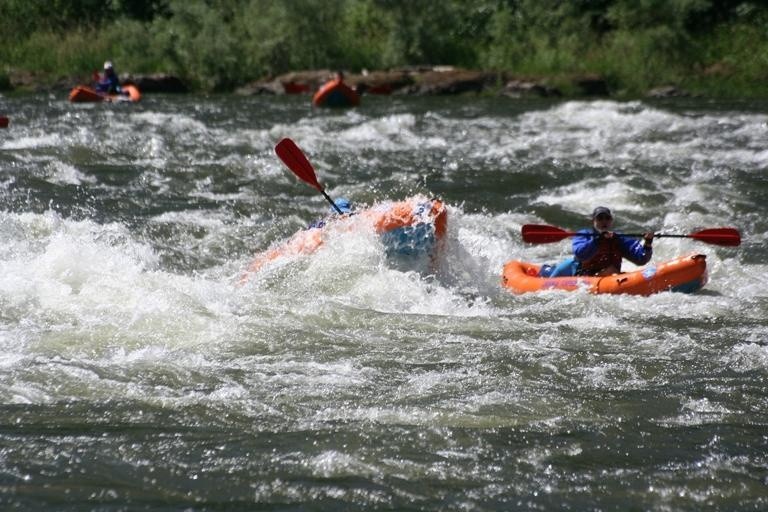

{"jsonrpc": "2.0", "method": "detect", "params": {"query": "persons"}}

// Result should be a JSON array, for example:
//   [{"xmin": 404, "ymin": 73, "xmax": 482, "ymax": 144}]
[
  {"xmin": 97, "ymin": 60, "xmax": 122, "ymax": 96},
  {"xmin": 333, "ymin": 70, "xmax": 344, "ymax": 80},
  {"xmin": 572, "ymin": 206, "xmax": 656, "ymax": 279},
  {"xmin": 310, "ymin": 198, "xmax": 353, "ymax": 229}
]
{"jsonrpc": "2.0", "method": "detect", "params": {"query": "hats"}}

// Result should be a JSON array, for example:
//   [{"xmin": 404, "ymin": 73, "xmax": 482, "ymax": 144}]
[
  {"xmin": 103, "ymin": 62, "xmax": 113, "ymax": 70},
  {"xmin": 594, "ymin": 207, "xmax": 610, "ymax": 219},
  {"xmin": 333, "ymin": 198, "xmax": 352, "ymax": 213}
]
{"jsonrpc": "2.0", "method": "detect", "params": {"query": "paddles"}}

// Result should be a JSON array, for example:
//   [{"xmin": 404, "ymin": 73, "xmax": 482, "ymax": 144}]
[
  {"xmin": 522, "ymin": 223, "xmax": 740, "ymax": 247},
  {"xmin": 284, "ymin": 83, "xmax": 391, "ymax": 95},
  {"xmin": 275, "ymin": 137, "xmax": 343, "ymax": 214}
]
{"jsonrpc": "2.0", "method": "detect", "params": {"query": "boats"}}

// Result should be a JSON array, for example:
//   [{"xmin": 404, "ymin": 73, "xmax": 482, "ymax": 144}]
[
  {"xmin": 311, "ymin": 79, "xmax": 361, "ymax": 108},
  {"xmin": 231, "ymin": 194, "xmax": 448, "ymax": 289},
  {"xmin": 68, "ymin": 84, "xmax": 142, "ymax": 102},
  {"xmin": 496, "ymin": 252, "xmax": 708, "ymax": 298}
]
